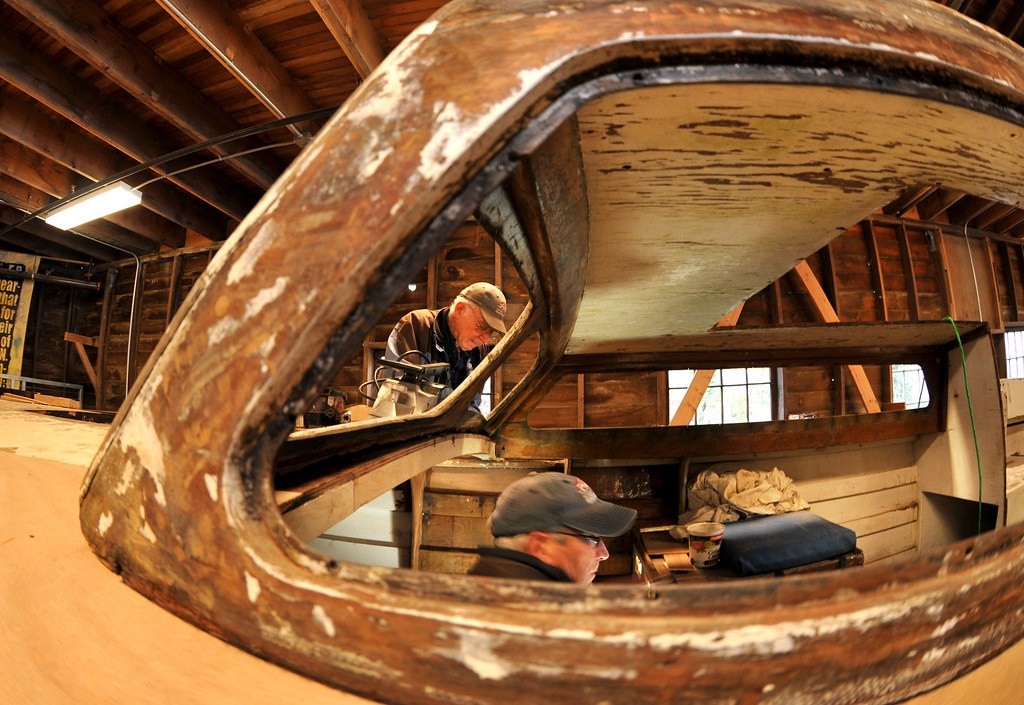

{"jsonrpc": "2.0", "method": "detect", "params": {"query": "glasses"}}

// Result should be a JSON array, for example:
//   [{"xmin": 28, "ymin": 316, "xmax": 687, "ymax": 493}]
[
  {"xmin": 470, "ymin": 306, "xmax": 503, "ymax": 341},
  {"xmin": 552, "ymin": 530, "xmax": 603, "ymax": 551}
]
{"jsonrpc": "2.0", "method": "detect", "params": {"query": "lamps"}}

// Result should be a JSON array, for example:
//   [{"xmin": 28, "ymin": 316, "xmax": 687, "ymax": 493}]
[{"xmin": 45, "ymin": 181, "xmax": 142, "ymax": 231}]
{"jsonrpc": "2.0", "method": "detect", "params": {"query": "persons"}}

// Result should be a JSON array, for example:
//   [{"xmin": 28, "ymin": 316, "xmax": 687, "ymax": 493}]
[
  {"xmin": 466, "ymin": 472, "xmax": 638, "ymax": 584},
  {"xmin": 368, "ymin": 282, "xmax": 507, "ymax": 413}
]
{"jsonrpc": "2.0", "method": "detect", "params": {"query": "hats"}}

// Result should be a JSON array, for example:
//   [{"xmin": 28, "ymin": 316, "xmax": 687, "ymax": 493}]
[
  {"xmin": 488, "ymin": 471, "xmax": 638, "ymax": 538},
  {"xmin": 460, "ymin": 282, "xmax": 507, "ymax": 336}
]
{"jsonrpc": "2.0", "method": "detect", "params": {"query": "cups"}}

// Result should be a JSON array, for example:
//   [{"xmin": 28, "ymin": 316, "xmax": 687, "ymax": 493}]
[{"xmin": 687, "ymin": 523, "xmax": 725, "ymax": 568}]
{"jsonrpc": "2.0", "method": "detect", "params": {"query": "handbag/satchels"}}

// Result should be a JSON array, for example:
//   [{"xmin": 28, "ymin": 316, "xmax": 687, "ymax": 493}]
[{"xmin": 712, "ymin": 509, "xmax": 858, "ymax": 578}]
